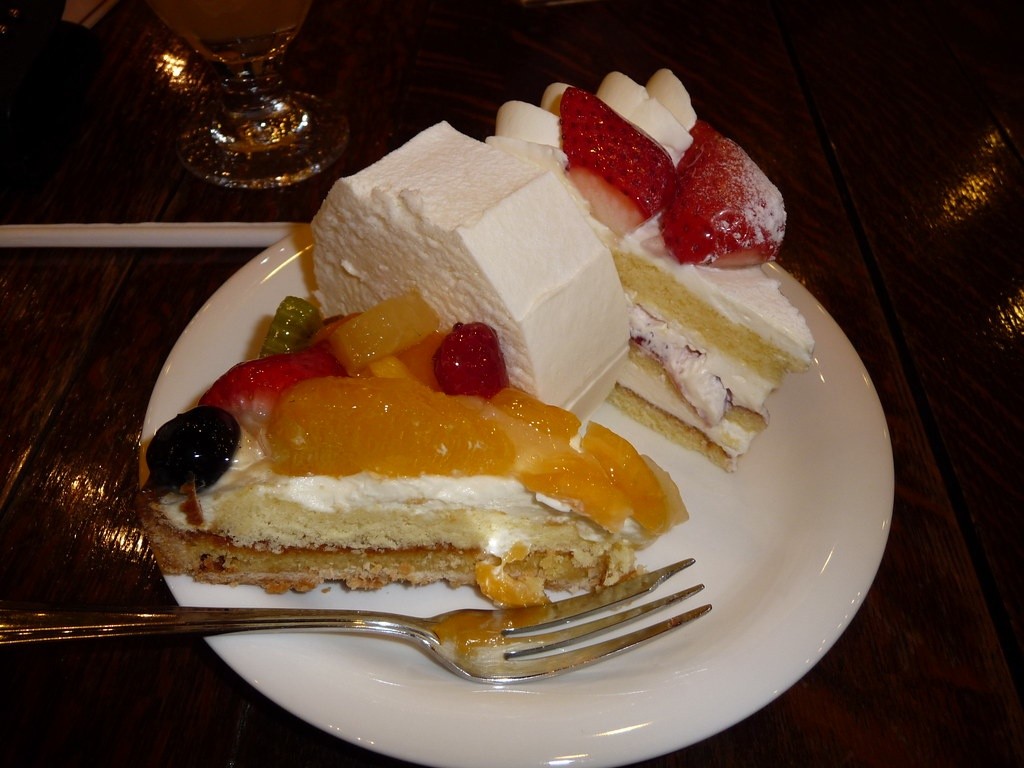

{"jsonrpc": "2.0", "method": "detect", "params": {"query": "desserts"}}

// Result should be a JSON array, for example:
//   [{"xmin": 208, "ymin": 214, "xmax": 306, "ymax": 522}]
[{"xmin": 132, "ymin": 69, "xmax": 814, "ymax": 609}]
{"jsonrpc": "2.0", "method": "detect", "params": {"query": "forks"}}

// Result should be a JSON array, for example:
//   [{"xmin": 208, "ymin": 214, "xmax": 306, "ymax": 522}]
[{"xmin": 0, "ymin": 558, "xmax": 713, "ymax": 688}]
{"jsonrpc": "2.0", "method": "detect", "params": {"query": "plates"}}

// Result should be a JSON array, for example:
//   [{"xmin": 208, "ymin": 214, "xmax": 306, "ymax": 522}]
[{"xmin": 134, "ymin": 213, "xmax": 896, "ymax": 768}]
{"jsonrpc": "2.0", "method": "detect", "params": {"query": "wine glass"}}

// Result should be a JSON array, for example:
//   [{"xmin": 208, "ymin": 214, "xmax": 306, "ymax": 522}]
[{"xmin": 133, "ymin": 0, "xmax": 347, "ymax": 192}]
